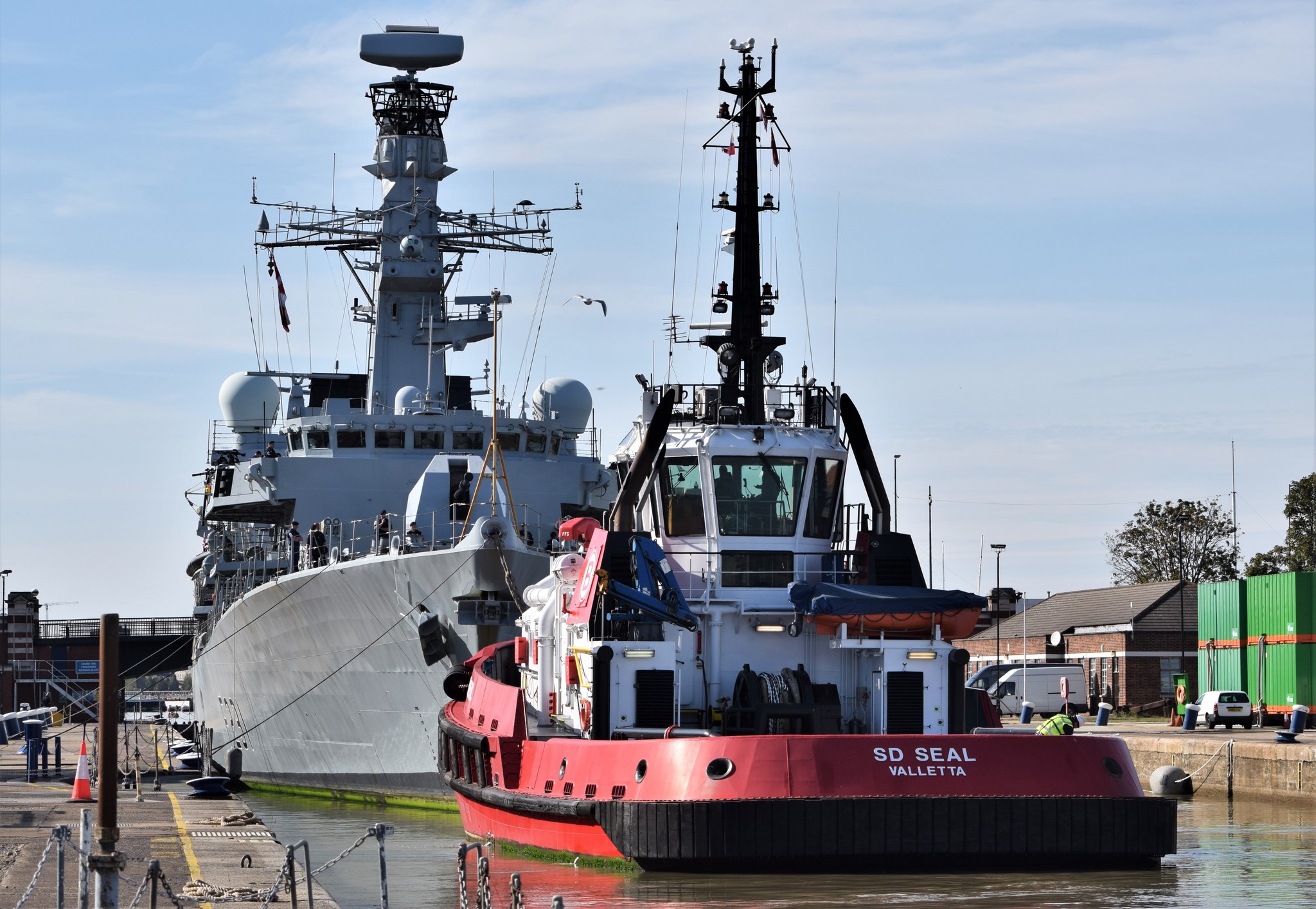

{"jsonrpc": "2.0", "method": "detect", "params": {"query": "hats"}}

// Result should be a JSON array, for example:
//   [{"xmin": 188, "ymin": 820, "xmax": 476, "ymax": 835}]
[
  {"xmin": 550, "ymin": 532, "xmax": 556, "ymax": 536},
  {"xmin": 293, "ymin": 521, "xmax": 299, "ymax": 525},
  {"xmin": 256, "ymin": 451, "xmax": 261, "ymax": 455},
  {"xmin": 410, "ymin": 522, "xmax": 416, "ymax": 526},
  {"xmin": 269, "ymin": 441, "xmax": 275, "ymax": 444},
  {"xmin": 380, "ymin": 510, "xmax": 387, "ymax": 515},
  {"xmin": 311, "ymin": 525, "xmax": 316, "ymax": 530}
]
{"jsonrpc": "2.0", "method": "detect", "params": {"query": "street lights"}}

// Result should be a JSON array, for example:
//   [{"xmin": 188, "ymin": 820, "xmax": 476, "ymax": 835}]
[
  {"xmin": 893, "ymin": 454, "xmax": 901, "ymax": 531},
  {"xmin": 0, "ymin": 569, "xmax": 12, "ymax": 715},
  {"xmin": 989, "ymin": 544, "xmax": 1006, "ymax": 722}
]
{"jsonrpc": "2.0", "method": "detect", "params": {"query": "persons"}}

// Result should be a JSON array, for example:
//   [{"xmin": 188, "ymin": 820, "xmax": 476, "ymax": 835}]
[
  {"xmin": 545, "ymin": 531, "xmax": 560, "ymax": 556},
  {"xmin": 1034, "ymin": 714, "xmax": 1085, "ymax": 736},
  {"xmin": 519, "ymin": 523, "xmax": 534, "ymax": 546},
  {"xmin": 286, "ymin": 521, "xmax": 304, "ymax": 573},
  {"xmin": 714, "ymin": 465, "xmax": 729, "ymax": 496},
  {"xmin": 373, "ymin": 509, "xmax": 393, "ymax": 540},
  {"xmin": 755, "ymin": 464, "xmax": 781, "ymax": 534},
  {"xmin": 305, "ymin": 524, "xmax": 329, "ymax": 569},
  {"xmin": 406, "ymin": 522, "xmax": 424, "ymax": 540},
  {"xmin": 248, "ymin": 441, "xmax": 281, "ymax": 461}
]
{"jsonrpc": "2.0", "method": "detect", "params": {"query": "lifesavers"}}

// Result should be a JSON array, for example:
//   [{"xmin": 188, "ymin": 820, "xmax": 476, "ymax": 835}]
[
  {"xmin": 1061, "ymin": 680, "xmax": 1064, "ymax": 693},
  {"xmin": 580, "ymin": 700, "xmax": 591, "ymax": 731},
  {"xmin": 1177, "ymin": 685, "xmax": 1185, "ymax": 703}
]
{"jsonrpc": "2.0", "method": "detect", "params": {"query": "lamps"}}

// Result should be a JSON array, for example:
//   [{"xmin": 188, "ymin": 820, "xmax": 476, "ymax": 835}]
[
  {"xmin": 754, "ymin": 426, "xmax": 764, "ymax": 440},
  {"xmin": 749, "ymin": 617, "xmax": 786, "ymax": 632},
  {"xmin": 332, "ymin": 517, "xmax": 341, "ymax": 534},
  {"xmin": 718, "ymin": 343, "xmax": 739, "ymax": 365},
  {"xmin": 635, "ymin": 374, "xmax": 647, "ymax": 388},
  {"xmin": 907, "ymin": 652, "xmax": 937, "ymax": 660},
  {"xmin": 717, "ymin": 406, "xmax": 740, "ymax": 426},
  {"xmin": 623, "ymin": 646, "xmax": 655, "ymax": 658},
  {"xmin": 713, "ymin": 299, "xmax": 728, "ymax": 313},
  {"xmin": 761, "ymin": 301, "xmax": 774, "ymax": 316},
  {"xmin": 437, "ymin": 391, "xmax": 444, "ymax": 407},
  {"xmin": 324, "ymin": 517, "xmax": 332, "ymax": 534},
  {"xmin": 763, "ymin": 350, "xmax": 783, "ymax": 388},
  {"xmin": 515, "ymin": 616, "xmax": 525, "ymax": 627},
  {"xmin": 772, "ymin": 408, "xmax": 795, "ymax": 426},
  {"xmin": 669, "ymin": 472, "xmax": 685, "ymax": 492},
  {"xmin": 373, "ymin": 391, "xmax": 380, "ymax": 406},
  {"xmin": 566, "ymin": 626, "xmax": 578, "ymax": 633}
]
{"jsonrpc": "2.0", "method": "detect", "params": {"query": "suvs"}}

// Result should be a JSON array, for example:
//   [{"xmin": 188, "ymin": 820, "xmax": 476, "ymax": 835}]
[{"xmin": 965, "ymin": 663, "xmax": 1086, "ymax": 697}]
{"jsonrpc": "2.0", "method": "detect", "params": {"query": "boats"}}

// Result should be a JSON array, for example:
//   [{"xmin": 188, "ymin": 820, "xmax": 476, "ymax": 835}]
[
  {"xmin": 430, "ymin": 32, "xmax": 1180, "ymax": 872},
  {"xmin": 182, "ymin": 17, "xmax": 624, "ymax": 817}
]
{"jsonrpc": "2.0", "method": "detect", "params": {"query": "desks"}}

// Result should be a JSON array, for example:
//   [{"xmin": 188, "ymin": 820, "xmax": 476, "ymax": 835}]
[{"xmin": 666, "ymin": 495, "xmax": 707, "ymax": 535}]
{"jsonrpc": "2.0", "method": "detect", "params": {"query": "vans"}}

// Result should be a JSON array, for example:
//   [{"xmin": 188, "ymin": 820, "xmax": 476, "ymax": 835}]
[{"xmin": 987, "ymin": 667, "xmax": 1090, "ymax": 718}]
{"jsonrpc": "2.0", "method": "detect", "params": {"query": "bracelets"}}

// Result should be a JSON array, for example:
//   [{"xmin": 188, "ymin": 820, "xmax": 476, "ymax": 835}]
[
  {"xmin": 307, "ymin": 552, "xmax": 309, "ymax": 554},
  {"xmin": 298, "ymin": 538, "xmax": 300, "ymax": 541}
]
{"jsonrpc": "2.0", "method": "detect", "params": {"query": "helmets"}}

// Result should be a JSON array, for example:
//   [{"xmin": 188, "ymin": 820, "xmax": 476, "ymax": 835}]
[{"xmin": 1074, "ymin": 714, "xmax": 1085, "ymax": 728}]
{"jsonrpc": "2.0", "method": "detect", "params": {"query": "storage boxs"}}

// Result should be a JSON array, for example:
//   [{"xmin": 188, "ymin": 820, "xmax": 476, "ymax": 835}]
[
  {"xmin": 675, "ymin": 487, "xmax": 684, "ymax": 494},
  {"xmin": 686, "ymin": 489, "xmax": 701, "ymax": 495}
]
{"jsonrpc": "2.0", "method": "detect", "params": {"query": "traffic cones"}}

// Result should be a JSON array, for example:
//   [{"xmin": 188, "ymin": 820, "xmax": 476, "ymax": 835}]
[
  {"xmin": 66, "ymin": 739, "xmax": 97, "ymax": 803},
  {"xmin": 1283, "ymin": 709, "xmax": 1291, "ymax": 729},
  {"xmin": 1167, "ymin": 708, "xmax": 1176, "ymax": 727}
]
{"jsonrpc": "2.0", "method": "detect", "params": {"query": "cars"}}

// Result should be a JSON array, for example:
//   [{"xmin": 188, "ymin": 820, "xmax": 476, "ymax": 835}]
[{"xmin": 1196, "ymin": 691, "xmax": 1254, "ymax": 729}]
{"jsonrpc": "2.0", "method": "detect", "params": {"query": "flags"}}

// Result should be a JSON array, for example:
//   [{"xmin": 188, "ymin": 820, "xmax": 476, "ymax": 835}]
[
  {"xmin": 720, "ymin": 127, "xmax": 736, "ymax": 155},
  {"xmin": 272, "ymin": 254, "xmax": 290, "ymax": 332},
  {"xmin": 770, "ymin": 126, "xmax": 780, "ymax": 168},
  {"xmin": 201, "ymin": 535, "xmax": 206, "ymax": 553},
  {"xmin": 759, "ymin": 100, "xmax": 768, "ymax": 133}
]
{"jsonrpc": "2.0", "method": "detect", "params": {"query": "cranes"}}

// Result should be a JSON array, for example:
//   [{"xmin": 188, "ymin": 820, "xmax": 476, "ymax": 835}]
[{"xmin": 42, "ymin": 601, "xmax": 80, "ymax": 619}]
